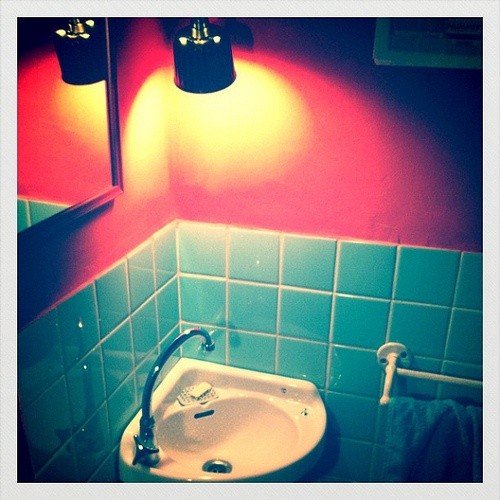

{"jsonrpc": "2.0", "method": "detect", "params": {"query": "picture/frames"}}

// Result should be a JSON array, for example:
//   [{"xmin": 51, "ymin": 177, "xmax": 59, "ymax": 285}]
[{"xmin": 372, "ymin": 17, "xmax": 483, "ymax": 70}]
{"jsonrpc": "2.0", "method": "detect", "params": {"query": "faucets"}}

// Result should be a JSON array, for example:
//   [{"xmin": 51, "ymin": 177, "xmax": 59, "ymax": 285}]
[{"xmin": 133, "ymin": 328, "xmax": 215, "ymax": 468}]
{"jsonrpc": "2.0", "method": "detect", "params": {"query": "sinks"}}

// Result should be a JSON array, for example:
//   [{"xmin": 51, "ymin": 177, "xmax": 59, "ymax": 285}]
[{"xmin": 119, "ymin": 358, "xmax": 329, "ymax": 480}]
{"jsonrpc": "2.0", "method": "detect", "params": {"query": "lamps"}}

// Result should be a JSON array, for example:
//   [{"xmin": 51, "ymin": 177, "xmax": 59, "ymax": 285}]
[
  {"xmin": 172, "ymin": 18, "xmax": 237, "ymax": 94},
  {"xmin": 53, "ymin": 17, "xmax": 107, "ymax": 85}
]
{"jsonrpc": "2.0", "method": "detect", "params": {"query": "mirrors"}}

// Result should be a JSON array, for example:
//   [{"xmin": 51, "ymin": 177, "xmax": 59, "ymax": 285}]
[{"xmin": 17, "ymin": 17, "xmax": 126, "ymax": 252}]
